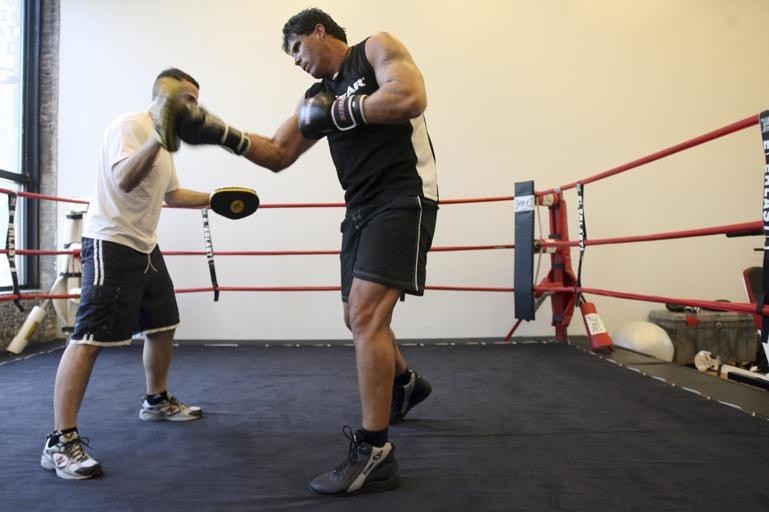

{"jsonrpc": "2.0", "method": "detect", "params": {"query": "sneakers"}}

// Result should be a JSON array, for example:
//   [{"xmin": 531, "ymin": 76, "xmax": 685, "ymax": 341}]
[
  {"xmin": 139, "ymin": 392, "xmax": 202, "ymax": 422},
  {"xmin": 309, "ymin": 425, "xmax": 400, "ymax": 497},
  {"xmin": 391, "ymin": 368, "xmax": 432, "ymax": 425},
  {"xmin": 41, "ymin": 429, "xmax": 103, "ymax": 480}
]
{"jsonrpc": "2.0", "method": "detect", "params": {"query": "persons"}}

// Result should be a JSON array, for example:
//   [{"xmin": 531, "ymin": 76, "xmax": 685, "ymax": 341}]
[
  {"xmin": 39, "ymin": 68, "xmax": 261, "ymax": 478},
  {"xmin": 148, "ymin": 8, "xmax": 440, "ymax": 498}
]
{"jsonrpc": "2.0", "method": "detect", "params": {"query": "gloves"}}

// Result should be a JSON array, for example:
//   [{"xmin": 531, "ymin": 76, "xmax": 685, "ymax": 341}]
[
  {"xmin": 176, "ymin": 103, "xmax": 251, "ymax": 158},
  {"xmin": 297, "ymin": 92, "xmax": 368, "ymax": 140}
]
